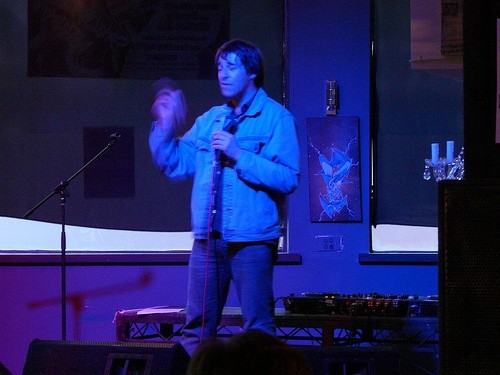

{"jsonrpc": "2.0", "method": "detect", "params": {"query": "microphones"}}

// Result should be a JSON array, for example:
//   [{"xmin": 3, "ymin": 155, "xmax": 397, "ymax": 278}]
[{"xmin": 212, "ymin": 149, "xmax": 220, "ymax": 169}]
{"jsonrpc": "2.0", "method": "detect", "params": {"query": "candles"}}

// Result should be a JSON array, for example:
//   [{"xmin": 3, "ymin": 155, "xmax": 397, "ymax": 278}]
[
  {"xmin": 431, "ymin": 142, "xmax": 440, "ymax": 162},
  {"xmin": 448, "ymin": 141, "xmax": 454, "ymax": 160}
]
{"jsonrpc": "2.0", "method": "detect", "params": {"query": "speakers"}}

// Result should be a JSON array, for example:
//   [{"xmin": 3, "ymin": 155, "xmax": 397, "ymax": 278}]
[
  {"xmin": 22, "ymin": 337, "xmax": 191, "ymax": 375},
  {"xmin": 438, "ymin": 178, "xmax": 500, "ymax": 375}
]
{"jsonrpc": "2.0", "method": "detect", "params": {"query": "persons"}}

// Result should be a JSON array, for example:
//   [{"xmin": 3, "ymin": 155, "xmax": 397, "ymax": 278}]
[
  {"xmin": 186, "ymin": 330, "xmax": 312, "ymax": 375},
  {"xmin": 148, "ymin": 39, "xmax": 301, "ymax": 358}
]
